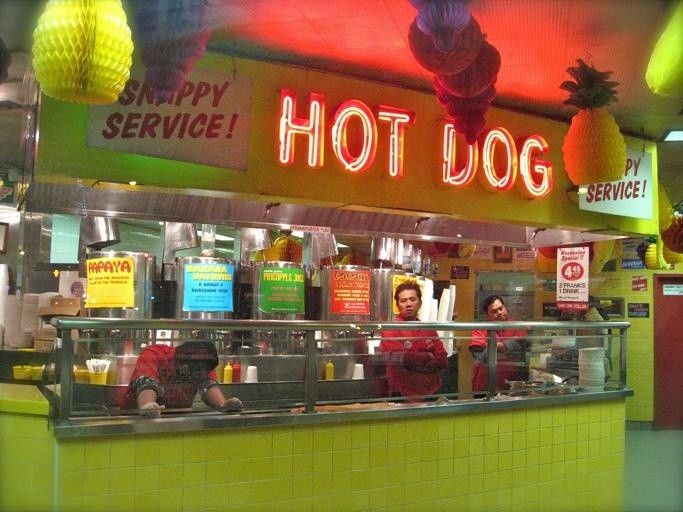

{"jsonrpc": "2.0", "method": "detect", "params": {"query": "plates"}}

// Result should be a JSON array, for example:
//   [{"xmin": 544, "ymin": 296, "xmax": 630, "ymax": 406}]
[{"xmin": 3, "ymin": 291, "xmax": 66, "ymax": 356}]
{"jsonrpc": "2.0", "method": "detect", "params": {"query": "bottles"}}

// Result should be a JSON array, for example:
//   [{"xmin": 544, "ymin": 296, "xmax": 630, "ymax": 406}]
[
  {"xmin": 325, "ymin": 358, "xmax": 334, "ymax": 380},
  {"xmin": 231, "ymin": 360, "xmax": 241, "ymax": 383},
  {"xmin": 223, "ymin": 361, "xmax": 233, "ymax": 384}
]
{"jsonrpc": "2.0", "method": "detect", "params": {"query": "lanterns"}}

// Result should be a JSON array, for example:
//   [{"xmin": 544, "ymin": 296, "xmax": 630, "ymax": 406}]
[
  {"xmin": 561, "ymin": 57, "xmax": 628, "ymax": 188},
  {"xmin": 130, "ymin": 0, "xmax": 212, "ymax": 106},
  {"xmin": 31, "ymin": 0, "xmax": 133, "ymax": 105},
  {"xmin": 407, "ymin": 0, "xmax": 497, "ymax": 144},
  {"xmin": 637, "ymin": 196, "xmax": 683, "ymax": 270}
]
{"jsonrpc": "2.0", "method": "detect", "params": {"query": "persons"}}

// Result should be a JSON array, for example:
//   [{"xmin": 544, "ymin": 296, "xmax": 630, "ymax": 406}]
[
  {"xmin": 576, "ymin": 298, "xmax": 608, "ymax": 355},
  {"xmin": 118, "ymin": 340, "xmax": 243, "ymax": 418},
  {"xmin": 379, "ymin": 279, "xmax": 450, "ymax": 402},
  {"xmin": 581, "ymin": 295, "xmax": 613, "ymax": 380},
  {"xmin": 70, "ymin": 281, "xmax": 86, "ymax": 301},
  {"xmin": 468, "ymin": 295, "xmax": 529, "ymax": 399}
]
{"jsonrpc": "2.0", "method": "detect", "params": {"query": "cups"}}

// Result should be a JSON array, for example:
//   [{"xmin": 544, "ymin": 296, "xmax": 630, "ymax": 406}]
[
  {"xmin": 244, "ymin": 365, "xmax": 258, "ymax": 384},
  {"xmin": 351, "ymin": 363, "xmax": 365, "ymax": 380},
  {"xmin": 11, "ymin": 362, "xmax": 108, "ymax": 386}
]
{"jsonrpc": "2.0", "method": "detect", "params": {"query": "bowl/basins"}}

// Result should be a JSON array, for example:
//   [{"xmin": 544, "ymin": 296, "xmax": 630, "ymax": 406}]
[{"xmin": 576, "ymin": 346, "xmax": 607, "ymax": 393}]
